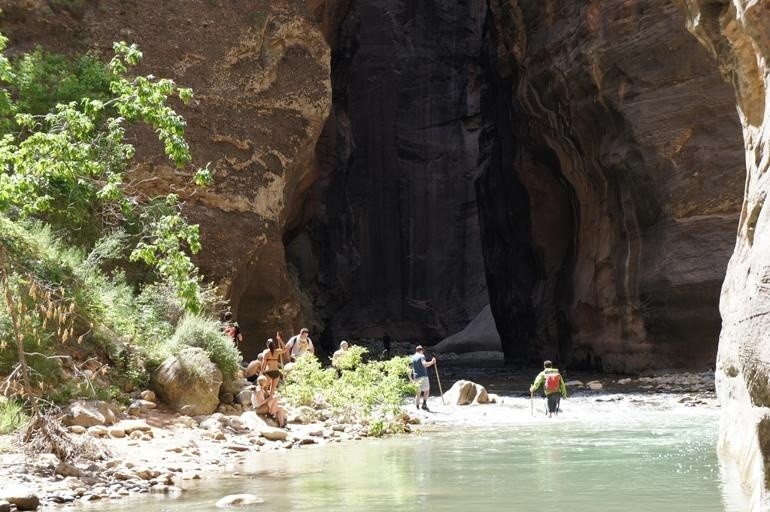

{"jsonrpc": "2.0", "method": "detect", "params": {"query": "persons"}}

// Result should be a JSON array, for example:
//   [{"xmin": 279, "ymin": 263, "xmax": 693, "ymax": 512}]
[
  {"xmin": 286, "ymin": 329, "xmax": 314, "ymax": 362},
  {"xmin": 331, "ymin": 341, "xmax": 348, "ymax": 363},
  {"xmin": 246, "ymin": 333, "xmax": 291, "ymax": 431},
  {"xmin": 407, "ymin": 345, "xmax": 436, "ymax": 411},
  {"xmin": 220, "ymin": 312, "xmax": 242, "ymax": 348},
  {"xmin": 529, "ymin": 360, "xmax": 567, "ymax": 413}
]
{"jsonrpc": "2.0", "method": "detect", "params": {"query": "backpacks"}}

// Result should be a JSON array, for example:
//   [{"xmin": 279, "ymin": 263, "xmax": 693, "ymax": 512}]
[{"xmin": 545, "ymin": 370, "xmax": 560, "ymax": 392}]
